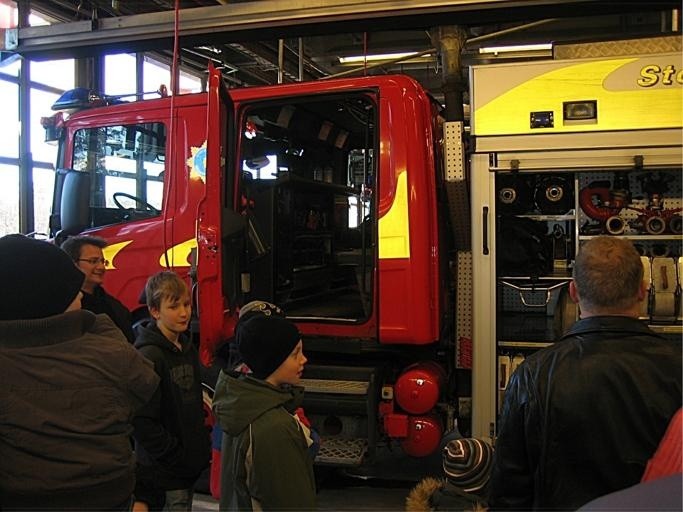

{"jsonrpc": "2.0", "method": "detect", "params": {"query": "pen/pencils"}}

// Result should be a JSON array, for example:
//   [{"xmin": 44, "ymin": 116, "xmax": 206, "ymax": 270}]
[{"xmin": 497, "ymin": 354, "xmax": 526, "ymax": 416}]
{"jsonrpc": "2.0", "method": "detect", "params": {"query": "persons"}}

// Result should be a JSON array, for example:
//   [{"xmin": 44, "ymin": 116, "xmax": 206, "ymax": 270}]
[
  {"xmin": 0, "ymin": 234, "xmax": 164, "ymax": 511},
  {"xmin": 207, "ymin": 299, "xmax": 321, "ymax": 501},
  {"xmin": 210, "ymin": 313, "xmax": 318, "ymax": 511},
  {"xmin": 59, "ymin": 235, "xmax": 135, "ymax": 340},
  {"xmin": 406, "ymin": 437, "xmax": 492, "ymax": 511},
  {"xmin": 126, "ymin": 270, "xmax": 206, "ymax": 512},
  {"xmin": 489, "ymin": 235, "xmax": 682, "ymax": 511}
]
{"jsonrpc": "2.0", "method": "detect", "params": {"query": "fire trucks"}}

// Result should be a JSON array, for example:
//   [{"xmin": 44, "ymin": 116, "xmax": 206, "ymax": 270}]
[{"xmin": 44, "ymin": 34, "xmax": 683, "ymax": 500}]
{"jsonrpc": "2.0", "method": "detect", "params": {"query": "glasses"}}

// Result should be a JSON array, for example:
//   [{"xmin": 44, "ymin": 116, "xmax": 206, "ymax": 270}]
[{"xmin": 79, "ymin": 257, "xmax": 109, "ymax": 266}]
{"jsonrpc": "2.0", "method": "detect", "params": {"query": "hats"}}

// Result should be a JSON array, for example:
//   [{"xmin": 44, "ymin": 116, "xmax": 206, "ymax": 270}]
[
  {"xmin": 442, "ymin": 438, "xmax": 495, "ymax": 492},
  {"xmin": 235, "ymin": 300, "xmax": 299, "ymax": 380},
  {"xmin": 0, "ymin": 234, "xmax": 85, "ymax": 320}
]
